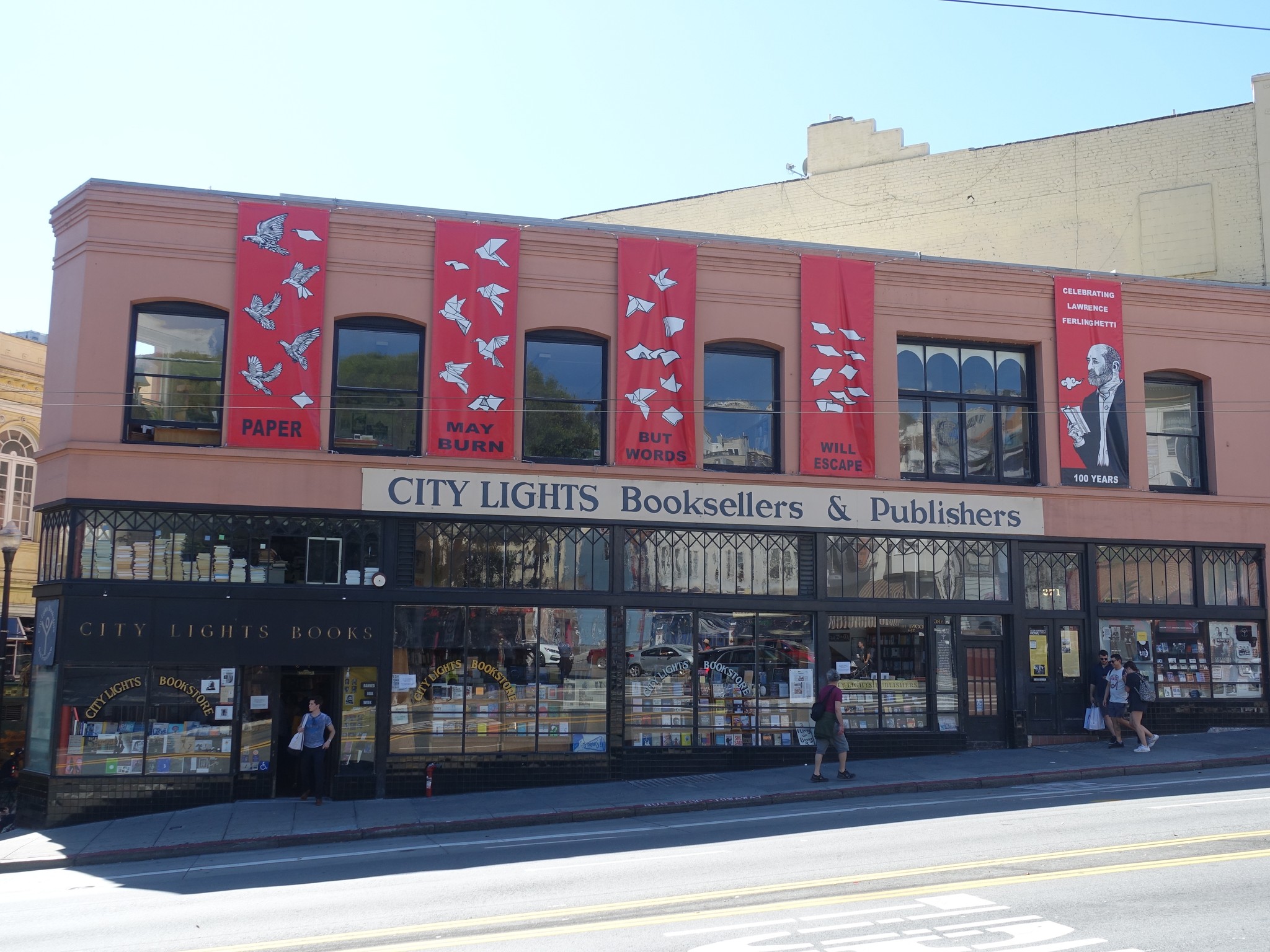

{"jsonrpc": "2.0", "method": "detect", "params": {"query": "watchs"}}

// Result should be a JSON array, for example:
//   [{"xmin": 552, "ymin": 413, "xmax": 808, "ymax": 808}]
[{"xmin": 328, "ymin": 739, "xmax": 331, "ymax": 742}]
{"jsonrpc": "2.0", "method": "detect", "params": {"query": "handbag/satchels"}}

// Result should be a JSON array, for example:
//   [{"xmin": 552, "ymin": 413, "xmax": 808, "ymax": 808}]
[
  {"xmin": 810, "ymin": 702, "xmax": 825, "ymax": 722},
  {"xmin": 1083, "ymin": 703, "xmax": 1105, "ymax": 731},
  {"xmin": 289, "ymin": 713, "xmax": 310, "ymax": 751}
]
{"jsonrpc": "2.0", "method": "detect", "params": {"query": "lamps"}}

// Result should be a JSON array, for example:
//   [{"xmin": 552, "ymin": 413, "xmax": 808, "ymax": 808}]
[
  {"xmin": 134, "ymin": 368, "xmax": 150, "ymax": 403},
  {"xmin": 372, "ymin": 572, "xmax": 386, "ymax": 587}
]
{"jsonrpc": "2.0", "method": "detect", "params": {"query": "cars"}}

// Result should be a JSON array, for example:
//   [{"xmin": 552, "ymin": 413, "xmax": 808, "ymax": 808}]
[
  {"xmin": 628, "ymin": 643, "xmax": 698, "ymax": 677},
  {"xmin": 697, "ymin": 645, "xmax": 816, "ymax": 696},
  {"xmin": 534, "ymin": 635, "xmax": 576, "ymax": 668},
  {"xmin": 741, "ymin": 638, "xmax": 815, "ymax": 670},
  {"xmin": 586, "ymin": 646, "xmax": 608, "ymax": 669}
]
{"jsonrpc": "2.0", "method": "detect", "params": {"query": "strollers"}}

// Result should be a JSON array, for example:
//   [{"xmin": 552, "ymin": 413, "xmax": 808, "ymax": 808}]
[{"xmin": 843, "ymin": 658, "xmax": 870, "ymax": 681}]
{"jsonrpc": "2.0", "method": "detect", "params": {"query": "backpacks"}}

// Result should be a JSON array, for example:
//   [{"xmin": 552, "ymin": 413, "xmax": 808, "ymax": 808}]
[{"xmin": 1132, "ymin": 672, "xmax": 1155, "ymax": 702}]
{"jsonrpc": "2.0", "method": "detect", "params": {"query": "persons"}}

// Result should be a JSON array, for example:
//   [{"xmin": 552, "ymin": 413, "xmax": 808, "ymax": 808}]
[
  {"xmin": 1212, "ymin": 627, "xmax": 1224, "ymax": 658},
  {"xmin": 1122, "ymin": 661, "xmax": 1159, "ymax": 752},
  {"xmin": 1111, "ymin": 641, "xmax": 1117, "ymax": 650},
  {"xmin": 1211, "ymin": 627, "xmax": 1233, "ymax": 659},
  {"xmin": 1124, "ymin": 625, "xmax": 1132, "ymax": 644},
  {"xmin": 1034, "ymin": 665, "xmax": 1045, "ymax": 675},
  {"xmin": 566, "ymin": 610, "xmax": 584, "ymax": 652},
  {"xmin": 810, "ymin": 669, "xmax": 856, "ymax": 782},
  {"xmin": 702, "ymin": 639, "xmax": 710, "ymax": 651},
  {"xmin": 1103, "ymin": 654, "xmax": 1142, "ymax": 749},
  {"xmin": 1089, "ymin": 650, "xmax": 1117, "ymax": 744},
  {"xmin": 856, "ymin": 641, "xmax": 870, "ymax": 677},
  {"xmin": 557, "ymin": 637, "xmax": 573, "ymax": 685},
  {"xmin": 297, "ymin": 697, "xmax": 335, "ymax": 805},
  {"xmin": 656, "ymin": 635, "xmax": 662, "ymax": 645}
]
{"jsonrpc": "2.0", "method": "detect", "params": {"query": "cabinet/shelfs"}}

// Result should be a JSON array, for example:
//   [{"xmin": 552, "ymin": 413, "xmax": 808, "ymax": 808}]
[
  {"xmin": 67, "ymin": 721, "xmax": 229, "ymax": 774},
  {"xmin": 427, "ymin": 674, "xmax": 927, "ymax": 751},
  {"xmin": 1154, "ymin": 632, "xmax": 1211, "ymax": 698}
]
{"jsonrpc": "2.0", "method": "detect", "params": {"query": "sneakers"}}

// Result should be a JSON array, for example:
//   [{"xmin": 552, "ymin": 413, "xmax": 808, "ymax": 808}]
[
  {"xmin": 837, "ymin": 771, "xmax": 856, "ymax": 779},
  {"xmin": 1133, "ymin": 743, "xmax": 1151, "ymax": 752},
  {"xmin": 811, "ymin": 773, "xmax": 829, "ymax": 783},
  {"xmin": 1147, "ymin": 734, "xmax": 1160, "ymax": 748}
]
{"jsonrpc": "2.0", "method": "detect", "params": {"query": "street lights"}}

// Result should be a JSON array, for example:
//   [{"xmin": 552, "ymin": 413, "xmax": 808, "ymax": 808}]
[{"xmin": 0, "ymin": 520, "xmax": 20, "ymax": 678}]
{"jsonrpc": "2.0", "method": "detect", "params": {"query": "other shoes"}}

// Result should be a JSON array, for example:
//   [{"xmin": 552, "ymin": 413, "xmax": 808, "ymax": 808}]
[
  {"xmin": 301, "ymin": 790, "xmax": 310, "ymax": 801},
  {"xmin": 316, "ymin": 798, "xmax": 322, "ymax": 805},
  {"xmin": 1108, "ymin": 735, "xmax": 1117, "ymax": 744},
  {"xmin": 1108, "ymin": 741, "xmax": 1124, "ymax": 748},
  {"xmin": 1136, "ymin": 732, "xmax": 1142, "ymax": 744}
]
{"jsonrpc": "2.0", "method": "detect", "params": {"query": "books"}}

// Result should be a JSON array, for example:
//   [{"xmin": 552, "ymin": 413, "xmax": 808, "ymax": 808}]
[
  {"xmin": 432, "ymin": 683, "xmax": 926, "ymax": 746},
  {"xmin": 1156, "ymin": 642, "xmax": 1207, "ymax": 697}
]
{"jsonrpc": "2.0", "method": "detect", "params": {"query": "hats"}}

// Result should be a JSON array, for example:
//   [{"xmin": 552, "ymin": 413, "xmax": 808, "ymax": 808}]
[{"xmin": 702, "ymin": 638, "xmax": 710, "ymax": 646}]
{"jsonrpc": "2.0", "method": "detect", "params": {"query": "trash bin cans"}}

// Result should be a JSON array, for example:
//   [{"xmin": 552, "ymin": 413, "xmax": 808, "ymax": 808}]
[{"xmin": 1006, "ymin": 709, "xmax": 1028, "ymax": 748}]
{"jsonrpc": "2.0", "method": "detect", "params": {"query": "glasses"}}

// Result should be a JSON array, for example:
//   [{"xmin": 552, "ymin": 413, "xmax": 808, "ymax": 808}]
[
  {"xmin": 1109, "ymin": 659, "xmax": 1119, "ymax": 664},
  {"xmin": 1100, "ymin": 658, "xmax": 1108, "ymax": 661}
]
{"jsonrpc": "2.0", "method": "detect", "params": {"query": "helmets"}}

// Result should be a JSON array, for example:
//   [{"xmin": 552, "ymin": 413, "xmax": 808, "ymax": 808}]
[{"xmin": 15, "ymin": 746, "xmax": 25, "ymax": 756}]
